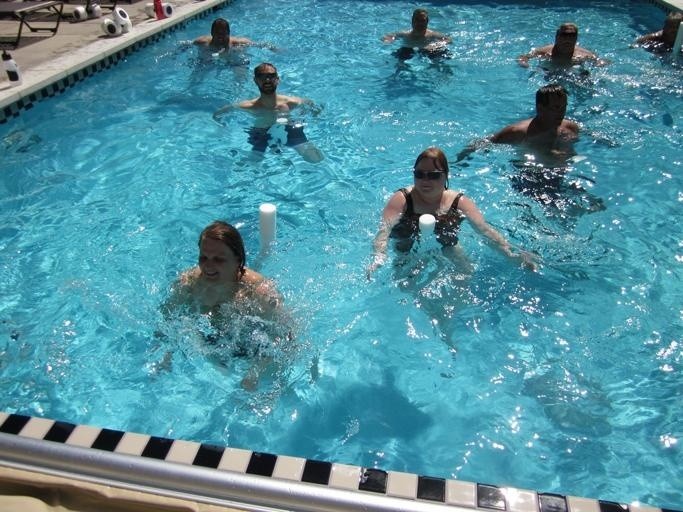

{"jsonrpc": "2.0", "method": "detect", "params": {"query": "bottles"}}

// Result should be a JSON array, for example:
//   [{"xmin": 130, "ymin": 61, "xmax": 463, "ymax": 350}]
[{"xmin": 1, "ymin": 48, "xmax": 23, "ymax": 86}]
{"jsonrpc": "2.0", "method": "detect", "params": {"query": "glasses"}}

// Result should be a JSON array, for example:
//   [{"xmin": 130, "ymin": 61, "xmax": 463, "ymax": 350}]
[
  {"xmin": 255, "ymin": 73, "xmax": 276, "ymax": 80},
  {"xmin": 413, "ymin": 170, "xmax": 444, "ymax": 179}
]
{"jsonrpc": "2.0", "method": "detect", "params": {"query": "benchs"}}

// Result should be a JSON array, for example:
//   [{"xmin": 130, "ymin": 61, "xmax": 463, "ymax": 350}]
[{"xmin": 0, "ymin": 0, "xmax": 65, "ymax": 50}]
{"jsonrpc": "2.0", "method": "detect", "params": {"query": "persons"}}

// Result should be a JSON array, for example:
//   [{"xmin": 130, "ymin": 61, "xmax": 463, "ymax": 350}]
[
  {"xmin": 179, "ymin": 18, "xmax": 285, "ymax": 87},
  {"xmin": 364, "ymin": 148, "xmax": 540, "ymax": 287},
  {"xmin": 150, "ymin": 222, "xmax": 293, "ymax": 390},
  {"xmin": 384, "ymin": 9, "xmax": 452, "ymax": 59},
  {"xmin": 629, "ymin": 10, "xmax": 682, "ymax": 52},
  {"xmin": 516, "ymin": 23, "xmax": 611, "ymax": 80},
  {"xmin": 460, "ymin": 85, "xmax": 619, "ymax": 214},
  {"xmin": 213, "ymin": 64, "xmax": 324, "ymax": 163}
]
